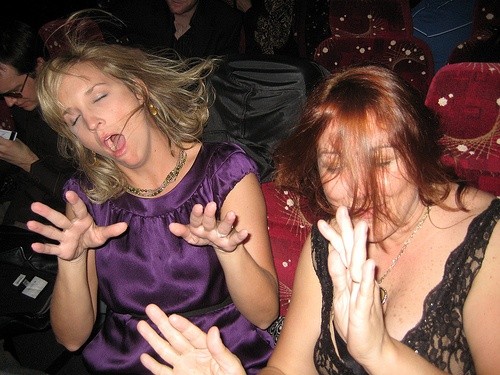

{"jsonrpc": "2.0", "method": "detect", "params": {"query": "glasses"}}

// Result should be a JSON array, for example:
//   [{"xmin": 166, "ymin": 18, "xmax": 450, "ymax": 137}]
[{"xmin": 0, "ymin": 69, "xmax": 30, "ymax": 99}]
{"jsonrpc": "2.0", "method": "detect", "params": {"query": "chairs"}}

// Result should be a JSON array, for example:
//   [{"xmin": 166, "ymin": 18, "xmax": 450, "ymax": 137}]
[{"xmin": 234, "ymin": 0, "xmax": 500, "ymax": 341}]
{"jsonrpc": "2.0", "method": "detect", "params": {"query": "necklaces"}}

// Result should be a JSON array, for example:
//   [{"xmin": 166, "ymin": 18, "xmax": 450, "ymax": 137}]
[
  {"xmin": 378, "ymin": 207, "xmax": 431, "ymax": 304},
  {"xmin": 127, "ymin": 149, "xmax": 186, "ymax": 197}
]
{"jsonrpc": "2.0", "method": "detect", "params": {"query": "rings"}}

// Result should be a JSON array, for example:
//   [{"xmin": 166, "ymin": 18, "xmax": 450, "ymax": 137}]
[{"xmin": 217, "ymin": 230, "xmax": 227, "ymax": 238}]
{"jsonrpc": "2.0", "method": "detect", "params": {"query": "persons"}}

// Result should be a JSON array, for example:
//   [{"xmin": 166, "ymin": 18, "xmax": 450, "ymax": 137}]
[
  {"xmin": 138, "ymin": 41, "xmax": 500, "ymax": 375},
  {"xmin": 128, "ymin": 0, "xmax": 241, "ymax": 91},
  {"xmin": 0, "ymin": 19, "xmax": 75, "ymax": 226},
  {"xmin": 27, "ymin": 26, "xmax": 279, "ymax": 375},
  {"xmin": 219, "ymin": 0, "xmax": 293, "ymax": 54}
]
